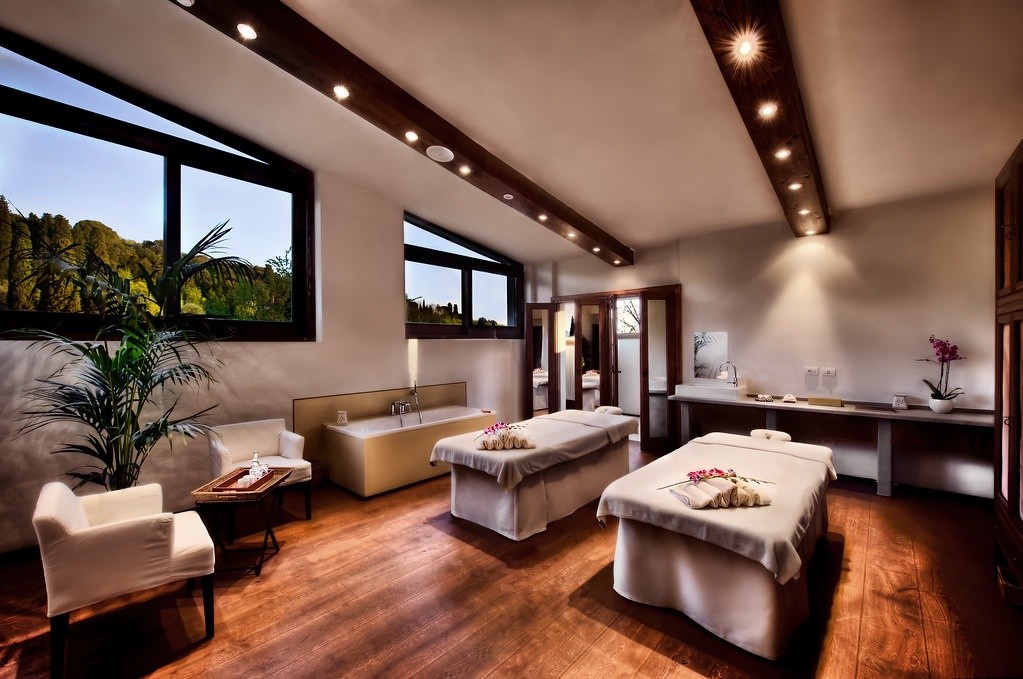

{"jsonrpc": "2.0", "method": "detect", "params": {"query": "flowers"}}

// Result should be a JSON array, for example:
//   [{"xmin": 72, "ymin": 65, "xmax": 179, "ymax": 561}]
[
  {"xmin": 657, "ymin": 467, "xmax": 776, "ymax": 491},
  {"xmin": 915, "ymin": 333, "xmax": 967, "ymax": 400},
  {"xmin": 586, "ymin": 369, "xmax": 600, "ymax": 376},
  {"xmin": 473, "ymin": 419, "xmax": 530, "ymax": 443},
  {"xmin": 533, "ymin": 368, "xmax": 547, "ymax": 374}
]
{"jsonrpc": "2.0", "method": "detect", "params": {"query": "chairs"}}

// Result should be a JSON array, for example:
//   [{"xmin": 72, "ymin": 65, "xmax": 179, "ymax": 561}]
[
  {"xmin": 205, "ymin": 419, "xmax": 312, "ymax": 544},
  {"xmin": 33, "ymin": 480, "xmax": 218, "ymax": 671}
]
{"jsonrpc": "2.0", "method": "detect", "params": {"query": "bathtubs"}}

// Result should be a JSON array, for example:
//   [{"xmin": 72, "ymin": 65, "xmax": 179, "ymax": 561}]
[{"xmin": 322, "ymin": 404, "xmax": 498, "ymax": 498}]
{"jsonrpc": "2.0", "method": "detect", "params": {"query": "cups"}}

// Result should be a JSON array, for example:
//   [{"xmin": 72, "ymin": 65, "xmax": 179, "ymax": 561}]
[
  {"xmin": 262, "ymin": 464, "xmax": 269, "ymax": 473},
  {"xmin": 243, "ymin": 475, "xmax": 254, "ymax": 484},
  {"xmin": 249, "ymin": 469, "xmax": 259, "ymax": 480},
  {"xmin": 238, "ymin": 478, "xmax": 249, "ymax": 488}
]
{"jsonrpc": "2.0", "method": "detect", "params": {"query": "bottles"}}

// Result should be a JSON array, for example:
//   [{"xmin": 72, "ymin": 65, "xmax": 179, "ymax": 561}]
[{"xmin": 251, "ymin": 451, "xmax": 263, "ymax": 478}]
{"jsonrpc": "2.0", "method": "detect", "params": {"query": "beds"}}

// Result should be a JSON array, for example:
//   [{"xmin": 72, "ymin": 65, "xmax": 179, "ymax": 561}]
[
  {"xmin": 429, "ymin": 406, "xmax": 638, "ymax": 549},
  {"xmin": 595, "ymin": 429, "xmax": 837, "ymax": 660}
]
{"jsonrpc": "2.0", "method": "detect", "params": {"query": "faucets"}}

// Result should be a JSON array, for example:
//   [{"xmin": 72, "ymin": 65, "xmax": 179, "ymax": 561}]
[
  {"xmin": 399, "ymin": 400, "xmax": 411, "ymax": 413},
  {"xmin": 719, "ymin": 361, "xmax": 738, "ymax": 388}
]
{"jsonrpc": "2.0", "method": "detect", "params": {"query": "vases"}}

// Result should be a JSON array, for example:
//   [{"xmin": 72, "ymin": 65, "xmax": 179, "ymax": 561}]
[{"xmin": 928, "ymin": 399, "xmax": 953, "ymax": 414}]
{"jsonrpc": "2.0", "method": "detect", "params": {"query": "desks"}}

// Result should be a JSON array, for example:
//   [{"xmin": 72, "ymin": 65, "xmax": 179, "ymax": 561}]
[
  {"xmin": 190, "ymin": 467, "xmax": 293, "ymax": 577},
  {"xmin": 667, "ymin": 394, "xmax": 995, "ymax": 497}
]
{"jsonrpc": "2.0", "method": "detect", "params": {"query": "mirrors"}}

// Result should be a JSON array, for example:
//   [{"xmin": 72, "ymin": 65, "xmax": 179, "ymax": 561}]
[{"xmin": 693, "ymin": 331, "xmax": 728, "ymax": 380}]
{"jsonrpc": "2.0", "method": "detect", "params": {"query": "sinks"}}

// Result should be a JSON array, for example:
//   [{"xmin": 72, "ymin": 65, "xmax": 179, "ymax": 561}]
[{"xmin": 675, "ymin": 381, "xmax": 746, "ymax": 400}]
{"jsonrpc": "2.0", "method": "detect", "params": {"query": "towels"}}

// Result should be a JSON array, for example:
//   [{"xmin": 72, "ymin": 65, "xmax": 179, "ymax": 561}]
[
  {"xmin": 670, "ymin": 474, "xmax": 771, "ymax": 511},
  {"xmin": 533, "ymin": 368, "xmax": 544, "ymax": 373},
  {"xmin": 475, "ymin": 428, "xmax": 536, "ymax": 451},
  {"xmin": 582, "ymin": 371, "xmax": 597, "ymax": 377}
]
